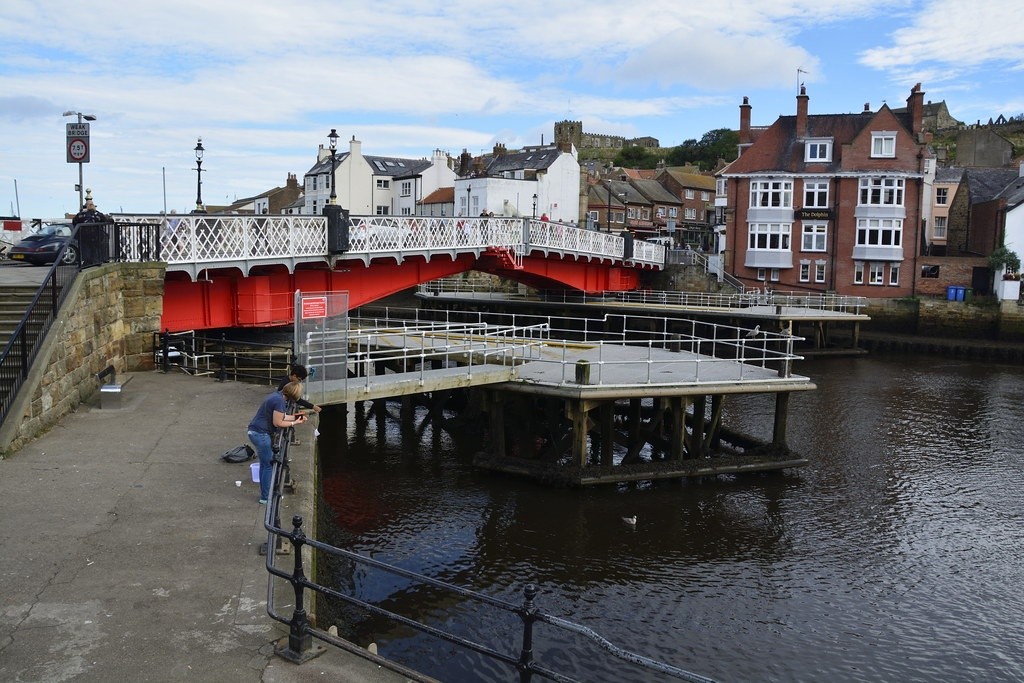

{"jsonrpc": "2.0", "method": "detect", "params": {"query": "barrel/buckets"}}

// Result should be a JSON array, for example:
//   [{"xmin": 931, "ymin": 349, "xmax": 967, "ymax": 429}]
[{"xmin": 250, "ymin": 463, "xmax": 260, "ymax": 482}]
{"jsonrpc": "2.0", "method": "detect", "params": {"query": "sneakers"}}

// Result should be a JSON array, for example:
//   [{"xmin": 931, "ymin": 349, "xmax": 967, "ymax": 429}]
[{"xmin": 260, "ymin": 496, "xmax": 276, "ymax": 505}]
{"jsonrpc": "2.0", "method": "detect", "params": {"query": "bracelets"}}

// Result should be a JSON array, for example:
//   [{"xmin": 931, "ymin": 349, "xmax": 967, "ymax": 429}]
[{"xmin": 291, "ymin": 421, "xmax": 293, "ymax": 427}]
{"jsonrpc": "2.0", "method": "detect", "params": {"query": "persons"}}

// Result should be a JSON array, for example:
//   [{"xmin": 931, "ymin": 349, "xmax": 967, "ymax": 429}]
[
  {"xmin": 567, "ymin": 220, "xmax": 577, "ymax": 228},
  {"xmin": 479, "ymin": 208, "xmax": 489, "ymax": 216},
  {"xmin": 558, "ymin": 218, "xmax": 564, "ymax": 225},
  {"xmin": 277, "ymin": 364, "xmax": 323, "ymax": 432},
  {"xmin": 541, "ymin": 213, "xmax": 549, "ymax": 222},
  {"xmin": 247, "ymin": 381, "xmax": 306, "ymax": 505}
]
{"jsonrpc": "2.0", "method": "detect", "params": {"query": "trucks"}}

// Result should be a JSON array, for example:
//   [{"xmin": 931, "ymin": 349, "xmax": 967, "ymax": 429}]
[{"xmin": 641, "ymin": 237, "xmax": 674, "ymax": 253}]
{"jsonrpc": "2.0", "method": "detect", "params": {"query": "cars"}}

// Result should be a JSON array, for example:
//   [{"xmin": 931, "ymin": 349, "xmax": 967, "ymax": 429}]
[
  {"xmin": 348, "ymin": 217, "xmax": 414, "ymax": 249},
  {"xmin": 7, "ymin": 223, "xmax": 110, "ymax": 266}
]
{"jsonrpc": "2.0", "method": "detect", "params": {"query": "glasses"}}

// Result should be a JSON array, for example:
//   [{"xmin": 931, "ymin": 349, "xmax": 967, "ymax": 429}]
[{"xmin": 295, "ymin": 376, "xmax": 301, "ymax": 382}]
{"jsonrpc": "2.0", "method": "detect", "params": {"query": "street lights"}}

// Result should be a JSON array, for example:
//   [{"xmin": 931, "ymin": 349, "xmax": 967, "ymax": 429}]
[
  {"xmin": 532, "ymin": 194, "xmax": 537, "ymax": 219},
  {"xmin": 327, "ymin": 129, "xmax": 340, "ymax": 205},
  {"xmin": 608, "ymin": 178, "xmax": 611, "ymax": 233},
  {"xmin": 62, "ymin": 110, "xmax": 97, "ymax": 212},
  {"xmin": 466, "ymin": 184, "xmax": 471, "ymax": 216},
  {"xmin": 622, "ymin": 199, "xmax": 629, "ymax": 231},
  {"xmin": 191, "ymin": 138, "xmax": 207, "ymax": 213}
]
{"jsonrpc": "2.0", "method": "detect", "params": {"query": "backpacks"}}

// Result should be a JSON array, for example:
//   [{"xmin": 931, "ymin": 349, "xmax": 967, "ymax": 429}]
[{"xmin": 221, "ymin": 443, "xmax": 255, "ymax": 463}]
